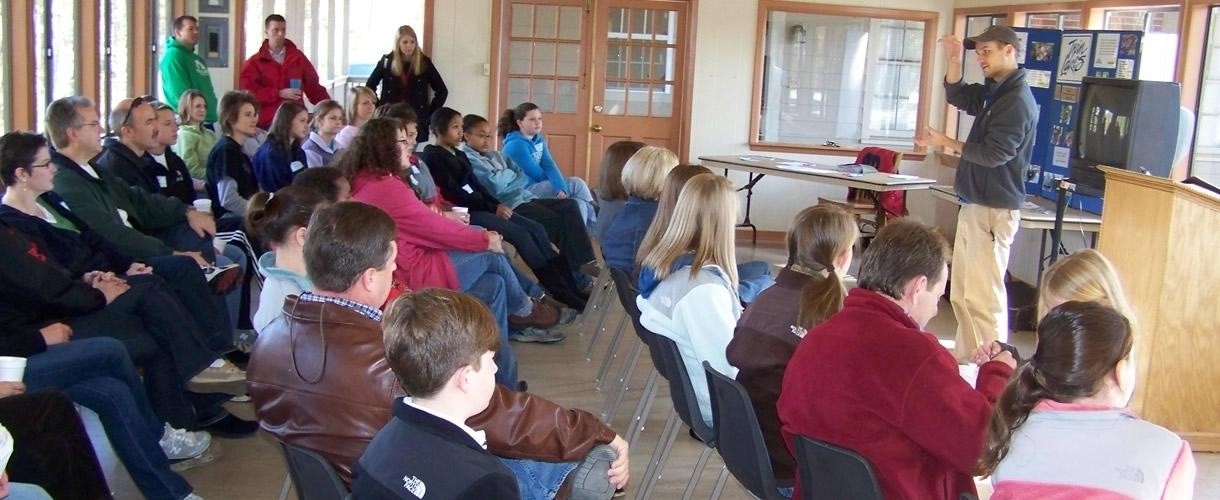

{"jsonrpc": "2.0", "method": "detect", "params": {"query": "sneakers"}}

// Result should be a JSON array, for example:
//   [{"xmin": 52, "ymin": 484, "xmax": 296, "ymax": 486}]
[
  {"xmin": 509, "ymin": 259, "xmax": 609, "ymax": 343},
  {"xmin": 159, "ymin": 317, "xmax": 259, "ymax": 500},
  {"xmin": 199, "ymin": 264, "xmax": 242, "ymax": 296}
]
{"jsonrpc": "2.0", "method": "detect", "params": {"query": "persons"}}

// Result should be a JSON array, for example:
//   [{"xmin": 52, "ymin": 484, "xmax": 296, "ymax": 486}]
[
  {"xmin": 725, "ymin": 203, "xmax": 860, "ymax": 495},
  {"xmin": 237, "ymin": 15, "xmax": 331, "ymax": 128},
  {"xmin": 972, "ymin": 300, "xmax": 1197, "ymax": 499},
  {"xmin": 787, "ymin": 221, "xmax": 1021, "ymax": 500},
  {"xmin": 350, "ymin": 287, "xmax": 520, "ymax": 500},
  {"xmin": 159, "ymin": 16, "xmax": 218, "ymax": 130},
  {"xmin": 246, "ymin": 201, "xmax": 631, "ymax": 499},
  {"xmin": 975, "ymin": 247, "xmax": 1122, "ymax": 365},
  {"xmin": 924, "ymin": 23, "xmax": 1039, "ymax": 366},
  {"xmin": 366, "ymin": 25, "xmax": 448, "ymax": 151},
  {"xmin": 594, "ymin": 141, "xmax": 775, "ymax": 427},
  {"xmin": 2, "ymin": 86, "xmax": 598, "ymax": 500}
]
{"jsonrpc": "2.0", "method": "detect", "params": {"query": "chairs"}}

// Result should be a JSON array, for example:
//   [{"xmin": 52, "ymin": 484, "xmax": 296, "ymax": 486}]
[
  {"xmin": 789, "ymin": 428, "xmax": 882, "ymax": 499},
  {"xmin": 700, "ymin": 360, "xmax": 792, "ymax": 498},
  {"xmin": 604, "ymin": 265, "xmax": 750, "ymax": 347},
  {"xmin": 631, "ymin": 308, "xmax": 751, "ymax": 449},
  {"xmin": 276, "ymin": 440, "xmax": 352, "ymax": 499}
]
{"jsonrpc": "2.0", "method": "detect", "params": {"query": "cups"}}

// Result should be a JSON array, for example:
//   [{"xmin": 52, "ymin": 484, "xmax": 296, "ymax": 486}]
[
  {"xmin": 290, "ymin": 79, "xmax": 302, "ymax": 90},
  {"xmin": 451, "ymin": 206, "xmax": 469, "ymax": 221},
  {"xmin": 193, "ymin": 198, "xmax": 212, "ymax": 213},
  {"xmin": 0, "ymin": 356, "xmax": 27, "ymax": 383}
]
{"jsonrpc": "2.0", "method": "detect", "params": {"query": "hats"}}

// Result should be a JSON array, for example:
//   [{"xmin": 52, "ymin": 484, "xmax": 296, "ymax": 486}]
[{"xmin": 963, "ymin": 25, "xmax": 1023, "ymax": 51}]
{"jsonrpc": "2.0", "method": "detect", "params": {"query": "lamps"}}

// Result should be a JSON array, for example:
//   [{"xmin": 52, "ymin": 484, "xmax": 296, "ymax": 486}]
[{"xmin": 792, "ymin": 24, "xmax": 808, "ymax": 44}]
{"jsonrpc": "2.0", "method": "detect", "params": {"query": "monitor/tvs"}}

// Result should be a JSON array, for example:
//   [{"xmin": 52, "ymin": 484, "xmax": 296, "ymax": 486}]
[{"xmin": 1070, "ymin": 76, "xmax": 1195, "ymax": 192}]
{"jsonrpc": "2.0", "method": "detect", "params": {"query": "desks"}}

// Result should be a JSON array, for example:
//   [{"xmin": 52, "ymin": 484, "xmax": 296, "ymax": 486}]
[
  {"xmin": 698, "ymin": 154, "xmax": 936, "ymax": 244},
  {"xmin": 929, "ymin": 184, "xmax": 1100, "ymax": 333}
]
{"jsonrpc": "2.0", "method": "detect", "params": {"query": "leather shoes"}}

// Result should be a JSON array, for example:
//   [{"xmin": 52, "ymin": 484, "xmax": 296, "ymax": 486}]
[{"xmin": 555, "ymin": 443, "xmax": 617, "ymax": 499}]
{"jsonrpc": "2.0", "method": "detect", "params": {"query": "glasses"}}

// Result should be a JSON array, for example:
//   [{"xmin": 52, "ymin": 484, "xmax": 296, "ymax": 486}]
[
  {"xmin": 123, "ymin": 97, "xmax": 143, "ymax": 125},
  {"xmin": 30, "ymin": 159, "xmax": 53, "ymax": 170}
]
